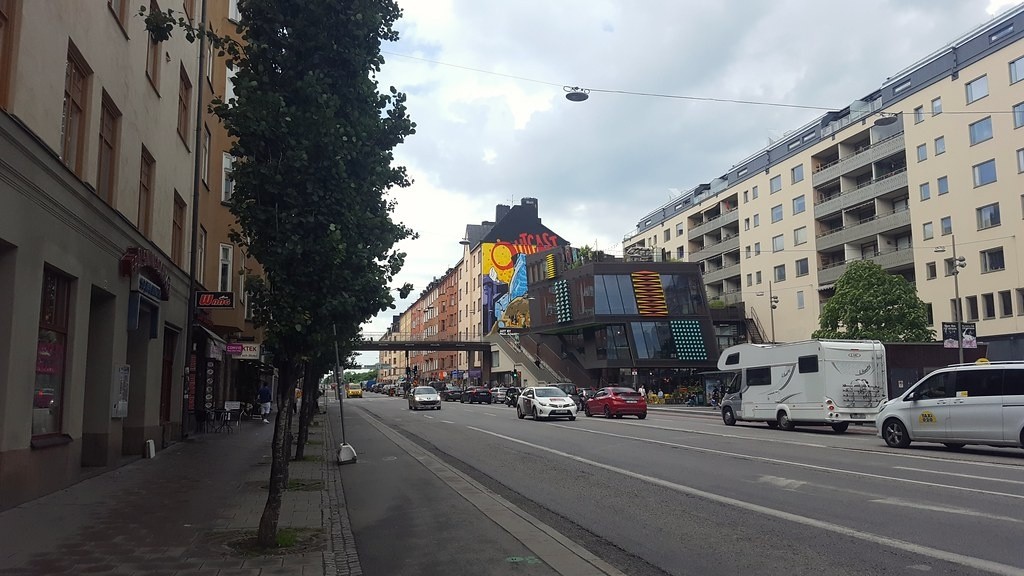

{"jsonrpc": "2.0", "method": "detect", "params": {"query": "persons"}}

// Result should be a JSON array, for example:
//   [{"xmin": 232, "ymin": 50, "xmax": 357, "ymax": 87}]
[
  {"xmin": 712, "ymin": 385, "xmax": 721, "ymax": 409},
  {"xmin": 637, "ymin": 383, "xmax": 664, "ymax": 405},
  {"xmin": 258, "ymin": 382, "xmax": 271, "ymax": 424},
  {"xmin": 687, "ymin": 391, "xmax": 696, "ymax": 404},
  {"xmin": 293, "ymin": 381, "xmax": 302, "ymax": 415}
]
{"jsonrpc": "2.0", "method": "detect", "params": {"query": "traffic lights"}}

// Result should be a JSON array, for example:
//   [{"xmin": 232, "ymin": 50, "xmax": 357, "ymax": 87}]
[{"xmin": 513, "ymin": 369, "xmax": 517, "ymax": 378}]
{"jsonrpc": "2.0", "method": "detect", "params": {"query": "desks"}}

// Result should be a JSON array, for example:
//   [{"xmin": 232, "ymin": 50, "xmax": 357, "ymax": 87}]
[{"xmin": 213, "ymin": 410, "xmax": 236, "ymax": 435}]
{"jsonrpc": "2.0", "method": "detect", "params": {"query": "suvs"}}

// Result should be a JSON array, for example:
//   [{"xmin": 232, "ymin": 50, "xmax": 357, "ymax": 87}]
[{"xmin": 875, "ymin": 360, "xmax": 1024, "ymax": 452}]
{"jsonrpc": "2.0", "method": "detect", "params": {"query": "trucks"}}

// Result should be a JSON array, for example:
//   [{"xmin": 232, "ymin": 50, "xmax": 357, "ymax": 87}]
[{"xmin": 719, "ymin": 338, "xmax": 889, "ymax": 434}]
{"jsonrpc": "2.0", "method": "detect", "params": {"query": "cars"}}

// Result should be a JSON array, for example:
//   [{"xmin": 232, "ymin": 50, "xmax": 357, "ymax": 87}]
[
  {"xmin": 439, "ymin": 385, "xmax": 464, "ymax": 401},
  {"xmin": 516, "ymin": 386, "xmax": 578, "ymax": 421},
  {"xmin": 361, "ymin": 379, "xmax": 446, "ymax": 397},
  {"xmin": 546, "ymin": 383, "xmax": 598, "ymax": 411},
  {"xmin": 460, "ymin": 386, "xmax": 491, "ymax": 404},
  {"xmin": 295, "ymin": 389, "xmax": 301, "ymax": 398},
  {"xmin": 346, "ymin": 382, "xmax": 363, "ymax": 398},
  {"xmin": 505, "ymin": 387, "xmax": 527, "ymax": 408},
  {"xmin": 490, "ymin": 387, "xmax": 509, "ymax": 404},
  {"xmin": 584, "ymin": 386, "xmax": 647, "ymax": 419},
  {"xmin": 408, "ymin": 386, "xmax": 442, "ymax": 411}
]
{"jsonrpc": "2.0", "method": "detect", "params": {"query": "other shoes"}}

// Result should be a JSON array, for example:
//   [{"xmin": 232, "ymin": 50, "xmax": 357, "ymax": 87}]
[{"xmin": 262, "ymin": 418, "xmax": 270, "ymax": 424}]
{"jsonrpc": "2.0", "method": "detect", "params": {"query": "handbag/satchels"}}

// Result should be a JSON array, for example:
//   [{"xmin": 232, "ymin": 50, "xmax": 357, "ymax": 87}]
[{"xmin": 711, "ymin": 400, "xmax": 717, "ymax": 406}]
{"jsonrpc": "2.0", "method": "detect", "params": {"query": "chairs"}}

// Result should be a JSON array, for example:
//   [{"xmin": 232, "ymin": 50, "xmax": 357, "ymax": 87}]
[
  {"xmin": 188, "ymin": 408, "xmax": 213, "ymax": 434},
  {"xmin": 220, "ymin": 409, "xmax": 244, "ymax": 431}
]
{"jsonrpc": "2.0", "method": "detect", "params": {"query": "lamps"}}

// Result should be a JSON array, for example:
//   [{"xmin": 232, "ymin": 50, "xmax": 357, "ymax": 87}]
[{"xmin": 566, "ymin": 90, "xmax": 589, "ymax": 101}]
